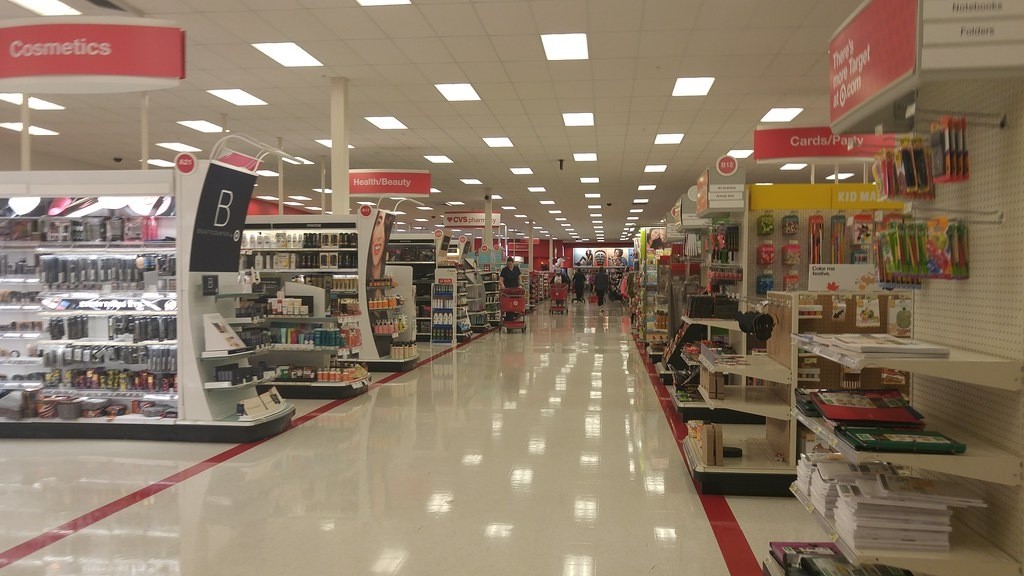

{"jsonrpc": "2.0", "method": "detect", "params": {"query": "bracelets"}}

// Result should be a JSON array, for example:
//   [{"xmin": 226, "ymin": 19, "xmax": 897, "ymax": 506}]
[{"xmin": 518, "ymin": 284, "xmax": 522, "ymax": 286}]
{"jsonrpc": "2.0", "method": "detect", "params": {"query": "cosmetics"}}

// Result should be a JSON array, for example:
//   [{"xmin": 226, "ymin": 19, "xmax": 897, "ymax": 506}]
[{"xmin": 233, "ymin": 232, "xmax": 454, "ymax": 385}]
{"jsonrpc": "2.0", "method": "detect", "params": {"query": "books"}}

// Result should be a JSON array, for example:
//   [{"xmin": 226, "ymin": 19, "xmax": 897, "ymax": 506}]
[
  {"xmin": 790, "ymin": 332, "xmax": 951, "ymax": 358},
  {"xmin": 794, "ymin": 452, "xmax": 989, "ymax": 553}
]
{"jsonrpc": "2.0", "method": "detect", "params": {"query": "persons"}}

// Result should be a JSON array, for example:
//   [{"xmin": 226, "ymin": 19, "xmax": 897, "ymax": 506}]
[
  {"xmin": 550, "ymin": 267, "xmax": 570, "ymax": 312},
  {"xmin": 589, "ymin": 273, "xmax": 595, "ymax": 293},
  {"xmin": 499, "ymin": 256, "xmax": 523, "ymax": 321},
  {"xmin": 646, "ymin": 228, "xmax": 664, "ymax": 250},
  {"xmin": 649, "ymin": 228, "xmax": 672, "ymax": 250},
  {"xmin": 365, "ymin": 211, "xmax": 396, "ymax": 288},
  {"xmin": 572, "ymin": 268, "xmax": 587, "ymax": 303},
  {"xmin": 593, "ymin": 267, "xmax": 607, "ymax": 306},
  {"xmin": 609, "ymin": 249, "xmax": 628, "ymax": 266},
  {"xmin": 574, "ymin": 249, "xmax": 593, "ymax": 266}
]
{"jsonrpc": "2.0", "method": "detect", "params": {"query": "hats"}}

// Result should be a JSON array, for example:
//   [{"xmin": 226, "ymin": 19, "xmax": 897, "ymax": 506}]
[{"xmin": 556, "ymin": 269, "xmax": 561, "ymax": 274}]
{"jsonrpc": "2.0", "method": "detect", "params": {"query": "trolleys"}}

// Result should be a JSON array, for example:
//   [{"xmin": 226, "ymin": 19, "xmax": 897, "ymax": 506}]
[
  {"xmin": 548, "ymin": 282, "xmax": 569, "ymax": 315},
  {"xmin": 497, "ymin": 285, "xmax": 527, "ymax": 335},
  {"xmin": 571, "ymin": 280, "xmax": 586, "ymax": 304}
]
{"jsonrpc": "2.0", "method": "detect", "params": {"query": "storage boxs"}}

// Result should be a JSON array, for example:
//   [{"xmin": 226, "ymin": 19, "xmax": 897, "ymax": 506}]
[
  {"xmin": 699, "ymin": 365, "xmax": 724, "ymax": 399},
  {"xmin": 699, "ymin": 423, "xmax": 724, "ymax": 467}
]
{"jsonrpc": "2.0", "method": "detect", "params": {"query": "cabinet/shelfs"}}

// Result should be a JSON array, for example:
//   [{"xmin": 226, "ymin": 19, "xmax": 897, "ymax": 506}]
[
  {"xmin": 763, "ymin": 67, "xmax": 1024, "ymax": 576},
  {"xmin": 686, "ymin": 181, "xmax": 916, "ymax": 499},
  {"xmin": 572, "ymin": 266, "xmax": 623, "ymax": 291},
  {"xmin": 668, "ymin": 194, "xmax": 766, "ymax": 425},
  {"xmin": 0, "ymin": 160, "xmax": 551, "ymax": 445},
  {"xmin": 628, "ymin": 182, "xmax": 746, "ymax": 384}
]
